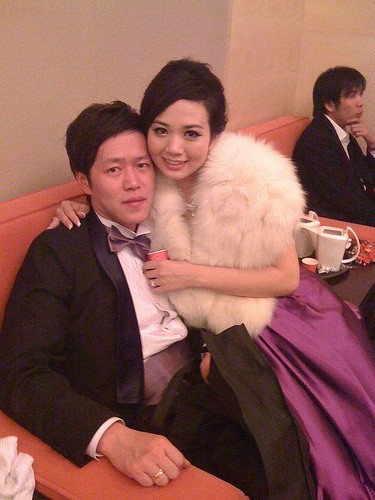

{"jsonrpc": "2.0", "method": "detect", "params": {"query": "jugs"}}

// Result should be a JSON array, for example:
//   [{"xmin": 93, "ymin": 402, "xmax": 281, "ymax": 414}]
[
  {"xmin": 302, "ymin": 225, "xmax": 360, "ymax": 272},
  {"xmin": 294, "ymin": 211, "xmax": 320, "ymax": 259}
]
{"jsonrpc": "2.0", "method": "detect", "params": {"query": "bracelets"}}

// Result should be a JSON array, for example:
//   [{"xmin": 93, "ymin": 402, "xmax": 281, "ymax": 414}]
[{"xmin": 367, "ymin": 146, "xmax": 375, "ymax": 151}]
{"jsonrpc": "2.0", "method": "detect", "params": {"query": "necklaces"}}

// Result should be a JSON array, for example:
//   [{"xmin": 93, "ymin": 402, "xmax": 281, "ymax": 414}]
[{"xmin": 187, "ymin": 201, "xmax": 197, "ymax": 217}]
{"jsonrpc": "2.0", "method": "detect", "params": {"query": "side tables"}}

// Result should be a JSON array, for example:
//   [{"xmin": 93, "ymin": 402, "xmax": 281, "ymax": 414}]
[{"xmin": 322, "ymin": 239, "xmax": 375, "ymax": 312}]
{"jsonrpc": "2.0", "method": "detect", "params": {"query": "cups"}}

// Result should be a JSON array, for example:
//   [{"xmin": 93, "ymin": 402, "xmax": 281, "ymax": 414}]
[
  {"xmin": 302, "ymin": 258, "xmax": 318, "ymax": 273},
  {"xmin": 147, "ymin": 250, "xmax": 169, "ymax": 261}
]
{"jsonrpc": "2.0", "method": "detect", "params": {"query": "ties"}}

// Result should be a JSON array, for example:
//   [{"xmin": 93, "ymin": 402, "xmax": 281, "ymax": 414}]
[
  {"xmin": 346, "ymin": 132, "xmax": 375, "ymax": 209},
  {"xmin": 101, "ymin": 222, "xmax": 153, "ymax": 261}
]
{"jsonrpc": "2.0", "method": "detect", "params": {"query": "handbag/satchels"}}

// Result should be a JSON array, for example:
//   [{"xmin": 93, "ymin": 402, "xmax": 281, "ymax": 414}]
[{"xmin": 150, "ymin": 324, "xmax": 317, "ymax": 500}]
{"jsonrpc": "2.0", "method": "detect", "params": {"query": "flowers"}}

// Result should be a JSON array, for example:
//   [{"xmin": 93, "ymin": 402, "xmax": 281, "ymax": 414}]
[{"xmin": 353, "ymin": 241, "xmax": 375, "ymax": 265}]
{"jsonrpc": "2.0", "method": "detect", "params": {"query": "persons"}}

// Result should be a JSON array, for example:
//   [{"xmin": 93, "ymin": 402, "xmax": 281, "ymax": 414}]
[
  {"xmin": 291, "ymin": 66, "xmax": 375, "ymax": 227},
  {"xmin": 1, "ymin": 101, "xmax": 316, "ymax": 500},
  {"xmin": 45, "ymin": 58, "xmax": 375, "ymax": 500}
]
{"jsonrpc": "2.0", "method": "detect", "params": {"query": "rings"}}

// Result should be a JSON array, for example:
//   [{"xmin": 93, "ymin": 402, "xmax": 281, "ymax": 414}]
[
  {"xmin": 151, "ymin": 469, "xmax": 163, "ymax": 480},
  {"xmin": 151, "ymin": 278, "xmax": 157, "ymax": 288}
]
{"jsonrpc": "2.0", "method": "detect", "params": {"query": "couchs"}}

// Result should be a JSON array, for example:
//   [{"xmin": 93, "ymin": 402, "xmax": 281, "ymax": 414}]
[{"xmin": 0, "ymin": 114, "xmax": 375, "ymax": 500}]
